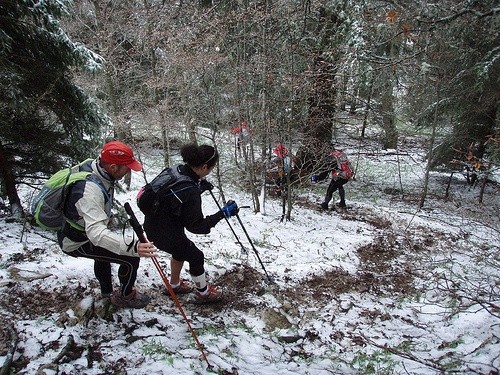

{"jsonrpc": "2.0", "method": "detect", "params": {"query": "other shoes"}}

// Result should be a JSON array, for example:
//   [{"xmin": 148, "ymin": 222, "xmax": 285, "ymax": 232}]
[
  {"xmin": 336, "ymin": 201, "xmax": 346, "ymax": 207},
  {"xmin": 316, "ymin": 202, "xmax": 328, "ymax": 209}
]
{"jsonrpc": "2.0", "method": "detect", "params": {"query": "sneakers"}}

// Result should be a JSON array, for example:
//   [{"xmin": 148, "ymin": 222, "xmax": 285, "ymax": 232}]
[
  {"xmin": 112, "ymin": 288, "xmax": 150, "ymax": 308},
  {"xmin": 193, "ymin": 285, "xmax": 222, "ymax": 303},
  {"xmin": 164, "ymin": 280, "xmax": 194, "ymax": 296}
]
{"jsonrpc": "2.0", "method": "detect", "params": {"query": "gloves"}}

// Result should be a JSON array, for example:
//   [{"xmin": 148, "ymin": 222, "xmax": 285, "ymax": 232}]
[
  {"xmin": 221, "ymin": 200, "xmax": 240, "ymax": 218},
  {"xmin": 311, "ymin": 175, "xmax": 318, "ymax": 183},
  {"xmin": 199, "ymin": 179, "xmax": 214, "ymax": 194}
]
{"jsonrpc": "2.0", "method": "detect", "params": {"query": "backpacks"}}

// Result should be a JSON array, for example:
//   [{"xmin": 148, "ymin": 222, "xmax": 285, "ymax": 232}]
[
  {"xmin": 31, "ymin": 159, "xmax": 110, "ymax": 232},
  {"xmin": 340, "ymin": 159, "xmax": 354, "ymax": 177},
  {"xmin": 137, "ymin": 167, "xmax": 199, "ymax": 219}
]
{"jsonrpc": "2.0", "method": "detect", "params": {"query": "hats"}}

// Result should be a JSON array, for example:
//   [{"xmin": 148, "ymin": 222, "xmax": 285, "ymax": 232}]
[{"xmin": 101, "ymin": 141, "xmax": 142, "ymax": 171}]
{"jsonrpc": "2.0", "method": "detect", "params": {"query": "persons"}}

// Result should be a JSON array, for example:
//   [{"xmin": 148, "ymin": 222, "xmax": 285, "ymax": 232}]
[
  {"xmin": 57, "ymin": 141, "xmax": 157, "ymax": 308},
  {"xmin": 316, "ymin": 145, "xmax": 354, "ymax": 209},
  {"xmin": 266, "ymin": 160, "xmax": 288, "ymax": 196},
  {"xmin": 143, "ymin": 143, "xmax": 240, "ymax": 303},
  {"xmin": 236, "ymin": 122, "xmax": 248, "ymax": 152}
]
